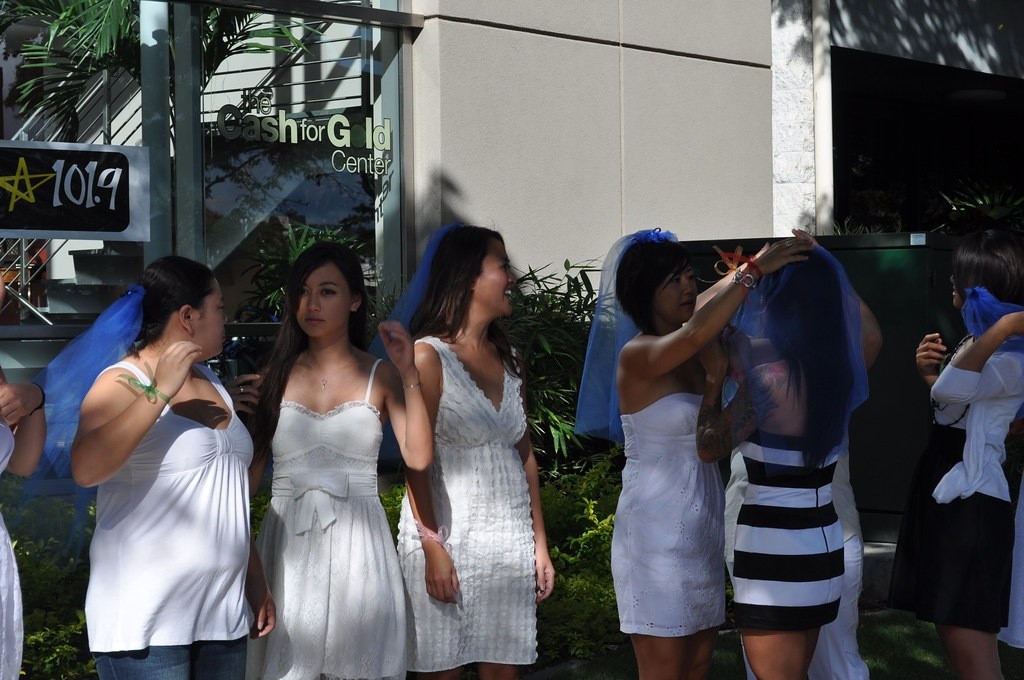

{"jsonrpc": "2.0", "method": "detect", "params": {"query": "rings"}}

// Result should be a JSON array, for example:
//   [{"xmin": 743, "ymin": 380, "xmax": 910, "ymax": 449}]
[
  {"xmin": 785, "ymin": 241, "xmax": 792, "ymax": 246},
  {"xmin": 240, "ymin": 386, "xmax": 244, "ymax": 392}
]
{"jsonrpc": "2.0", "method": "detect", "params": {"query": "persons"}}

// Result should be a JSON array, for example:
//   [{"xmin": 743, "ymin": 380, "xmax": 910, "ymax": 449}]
[
  {"xmin": 366, "ymin": 224, "xmax": 555, "ymax": 680},
  {"xmin": 221, "ymin": 240, "xmax": 434, "ymax": 680},
  {"xmin": 887, "ymin": 229, "xmax": 1024, "ymax": 680},
  {"xmin": 573, "ymin": 228, "xmax": 882, "ymax": 680},
  {"xmin": 0, "ymin": 275, "xmax": 48, "ymax": 680},
  {"xmin": 15, "ymin": 255, "xmax": 277, "ymax": 680}
]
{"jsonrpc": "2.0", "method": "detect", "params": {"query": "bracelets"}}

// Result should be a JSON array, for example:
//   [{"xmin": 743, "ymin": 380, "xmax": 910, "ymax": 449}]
[
  {"xmin": 724, "ymin": 253, "xmax": 763, "ymax": 303},
  {"xmin": 118, "ymin": 361, "xmax": 172, "ymax": 404},
  {"xmin": 402, "ymin": 383, "xmax": 421, "ymax": 389},
  {"xmin": 29, "ymin": 382, "xmax": 45, "ymax": 416},
  {"xmin": 411, "ymin": 519, "xmax": 448, "ymax": 548}
]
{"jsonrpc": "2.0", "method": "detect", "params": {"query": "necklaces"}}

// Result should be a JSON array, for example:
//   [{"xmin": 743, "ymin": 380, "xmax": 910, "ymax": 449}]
[{"xmin": 303, "ymin": 347, "xmax": 357, "ymax": 390}]
